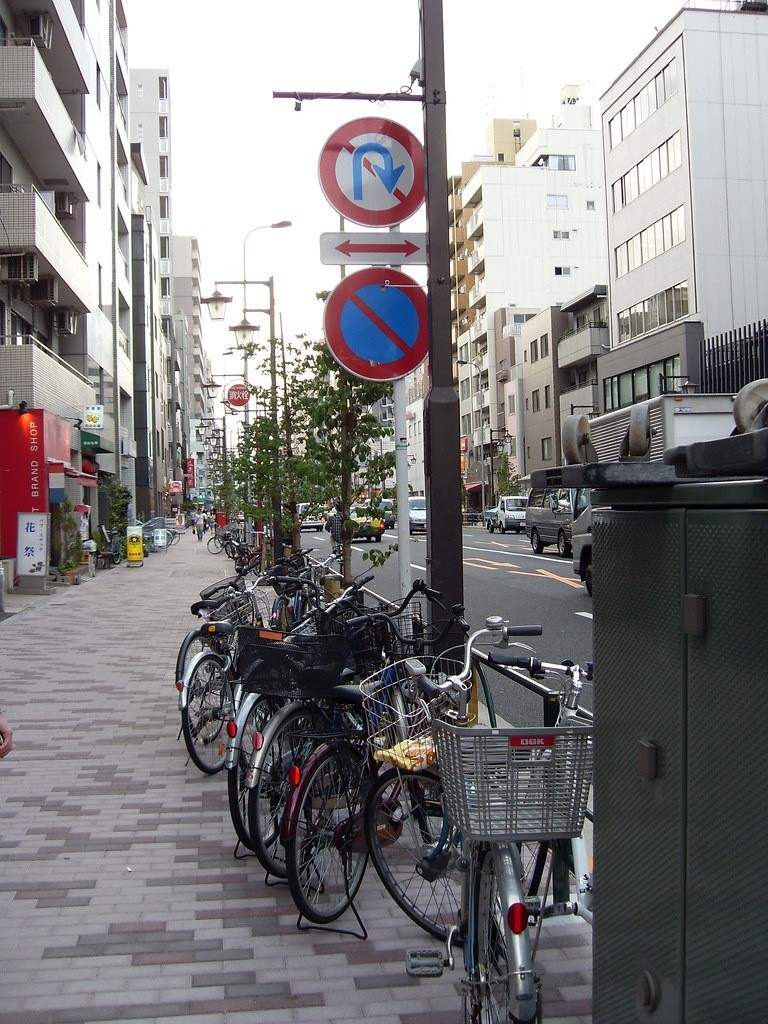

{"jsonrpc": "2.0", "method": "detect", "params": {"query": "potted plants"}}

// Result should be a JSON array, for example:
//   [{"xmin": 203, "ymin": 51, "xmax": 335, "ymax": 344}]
[{"xmin": 54, "ymin": 496, "xmax": 79, "ymax": 582}]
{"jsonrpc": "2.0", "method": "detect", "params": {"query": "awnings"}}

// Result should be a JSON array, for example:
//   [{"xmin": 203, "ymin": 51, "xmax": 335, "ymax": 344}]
[
  {"xmin": 463, "ymin": 483, "xmax": 482, "ymax": 493},
  {"xmin": 48, "ymin": 460, "xmax": 98, "ymax": 488}
]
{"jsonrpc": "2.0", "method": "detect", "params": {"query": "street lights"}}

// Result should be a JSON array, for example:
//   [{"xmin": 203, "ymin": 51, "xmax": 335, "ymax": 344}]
[
  {"xmin": 457, "ymin": 360, "xmax": 486, "ymax": 527},
  {"xmin": 571, "ymin": 405, "xmax": 601, "ymax": 420},
  {"xmin": 490, "ymin": 428, "xmax": 512, "ymax": 507},
  {"xmin": 241, "ymin": 219, "xmax": 292, "ymax": 540},
  {"xmin": 657, "ymin": 375, "xmax": 700, "ymax": 395},
  {"xmin": 203, "ymin": 281, "xmax": 283, "ymax": 573}
]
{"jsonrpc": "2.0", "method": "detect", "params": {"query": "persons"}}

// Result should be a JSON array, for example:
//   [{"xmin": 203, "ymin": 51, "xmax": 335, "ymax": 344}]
[
  {"xmin": 0, "ymin": 713, "xmax": 14, "ymax": 759},
  {"xmin": 325, "ymin": 502, "xmax": 351, "ymax": 558},
  {"xmin": 190, "ymin": 507, "xmax": 216, "ymax": 541}
]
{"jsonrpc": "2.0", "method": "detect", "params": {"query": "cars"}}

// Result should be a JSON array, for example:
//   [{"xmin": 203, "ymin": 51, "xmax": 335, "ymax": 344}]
[
  {"xmin": 350, "ymin": 507, "xmax": 385, "ymax": 542},
  {"xmin": 236, "ymin": 512, "xmax": 245, "ymax": 523},
  {"xmin": 321, "ymin": 505, "xmax": 354, "ymax": 520}
]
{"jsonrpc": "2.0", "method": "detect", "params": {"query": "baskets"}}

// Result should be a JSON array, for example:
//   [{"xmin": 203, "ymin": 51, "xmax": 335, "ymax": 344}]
[
  {"xmin": 201, "ymin": 577, "xmax": 247, "ymax": 619},
  {"xmin": 431, "ymin": 717, "xmax": 597, "ymax": 842},
  {"xmin": 268, "ymin": 557, "xmax": 308, "ymax": 595},
  {"xmin": 335, "ymin": 601, "xmax": 429, "ymax": 663},
  {"xmin": 359, "ymin": 657, "xmax": 471, "ymax": 773},
  {"xmin": 235, "ymin": 622, "xmax": 349, "ymax": 699}
]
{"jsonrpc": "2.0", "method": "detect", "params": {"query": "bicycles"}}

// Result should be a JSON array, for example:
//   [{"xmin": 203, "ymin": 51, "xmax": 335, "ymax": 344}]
[
  {"xmin": 137, "ymin": 518, "xmax": 181, "ymax": 548},
  {"xmin": 207, "ymin": 524, "xmax": 238, "ymax": 554},
  {"xmin": 174, "ymin": 540, "xmax": 597, "ymax": 1024},
  {"xmin": 90, "ymin": 527, "xmax": 127, "ymax": 568}
]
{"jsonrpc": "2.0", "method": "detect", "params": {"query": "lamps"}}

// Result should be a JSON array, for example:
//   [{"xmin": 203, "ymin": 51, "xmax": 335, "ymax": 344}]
[
  {"xmin": 63, "ymin": 417, "xmax": 84, "ymax": 430},
  {"xmin": 18, "ymin": 399, "xmax": 29, "ymax": 414}
]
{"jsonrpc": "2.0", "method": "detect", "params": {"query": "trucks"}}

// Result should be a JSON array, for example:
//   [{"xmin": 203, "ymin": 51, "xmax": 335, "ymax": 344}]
[
  {"xmin": 484, "ymin": 496, "xmax": 528, "ymax": 533},
  {"xmin": 548, "ymin": 393, "xmax": 737, "ymax": 595}
]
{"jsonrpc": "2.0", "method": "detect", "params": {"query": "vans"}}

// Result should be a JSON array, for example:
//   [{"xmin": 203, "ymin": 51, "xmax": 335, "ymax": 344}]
[
  {"xmin": 296, "ymin": 503, "xmax": 324, "ymax": 531},
  {"xmin": 407, "ymin": 496, "xmax": 427, "ymax": 534},
  {"xmin": 526, "ymin": 485, "xmax": 591, "ymax": 557},
  {"xmin": 364, "ymin": 499, "xmax": 396, "ymax": 529}
]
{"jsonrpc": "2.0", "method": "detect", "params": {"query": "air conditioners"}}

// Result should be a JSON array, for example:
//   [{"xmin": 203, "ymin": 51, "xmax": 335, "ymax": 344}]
[
  {"xmin": 22, "ymin": 279, "xmax": 60, "ymax": 307},
  {"xmin": 0, "ymin": 255, "xmax": 39, "ymax": 285},
  {"xmin": 25, "ymin": 14, "xmax": 51, "ymax": 49},
  {"xmin": 58, "ymin": 192, "xmax": 72, "ymax": 217},
  {"xmin": 50, "ymin": 310, "xmax": 74, "ymax": 336}
]
